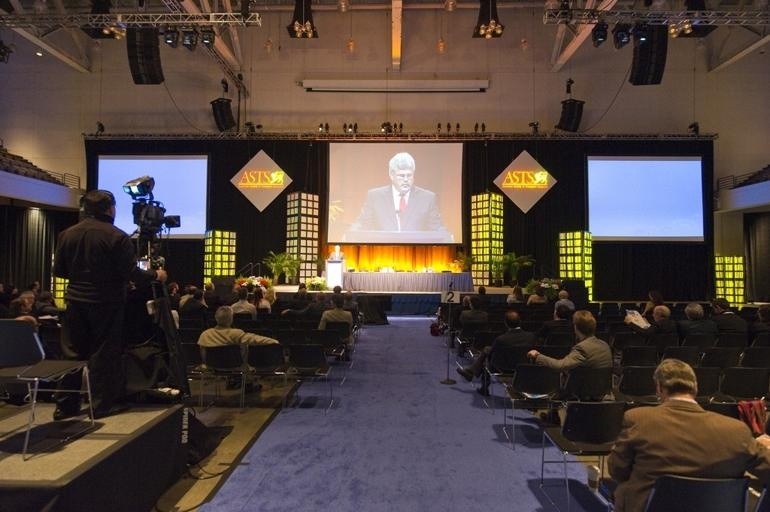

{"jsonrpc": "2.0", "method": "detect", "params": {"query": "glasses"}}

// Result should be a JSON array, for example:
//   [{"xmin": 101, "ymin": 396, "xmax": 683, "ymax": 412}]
[{"xmin": 397, "ymin": 173, "xmax": 414, "ymax": 180}]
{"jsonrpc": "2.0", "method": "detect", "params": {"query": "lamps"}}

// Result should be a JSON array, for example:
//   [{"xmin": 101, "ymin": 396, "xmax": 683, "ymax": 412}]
[
  {"xmin": 475, "ymin": 123, "xmax": 478, "ymax": 132},
  {"xmin": 381, "ymin": 0, "xmax": 393, "ymax": 138},
  {"xmin": 682, "ymin": 19, "xmax": 693, "ymax": 34},
  {"xmin": 393, "ymin": 124, "xmax": 398, "ymax": 133},
  {"xmin": 164, "ymin": 27, "xmax": 179, "ymax": 48},
  {"xmin": 669, "ymin": 24, "xmax": 679, "ymax": 38},
  {"xmin": 480, "ymin": 0, "xmax": 503, "ymax": 40},
  {"xmin": 347, "ymin": 0, "xmax": 355, "ymax": 54},
  {"xmin": 343, "ymin": 123, "xmax": 347, "ymax": 133},
  {"xmin": 529, "ymin": 0, "xmax": 539, "ymax": 134},
  {"xmin": 230, "ymin": 0, "xmax": 256, "ymax": 19},
  {"xmin": 354, "ymin": 123, "xmax": 358, "ymax": 135},
  {"xmin": 566, "ymin": 24, "xmax": 575, "ymax": 93},
  {"xmin": 522, "ymin": 39, "xmax": 528, "ymax": 50},
  {"xmin": 445, "ymin": 0, "xmax": 457, "ymax": 12},
  {"xmin": 456, "ymin": 123, "xmax": 460, "ymax": 133},
  {"xmin": 437, "ymin": 124, "xmax": 441, "ymax": 132},
  {"xmin": 482, "ymin": 122, "xmax": 485, "ymax": 133},
  {"xmin": 689, "ymin": 36, "xmax": 699, "ymax": 136},
  {"xmin": 611, "ymin": 22, "xmax": 633, "ymax": 49},
  {"xmin": 319, "ymin": 124, "xmax": 323, "ymax": 133},
  {"xmin": 210, "ymin": 71, "xmax": 236, "ymax": 133},
  {"xmin": 294, "ymin": 0, "xmax": 313, "ymax": 40},
  {"xmin": 181, "ymin": 27, "xmax": 199, "ymax": 52},
  {"xmin": 349, "ymin": 124, "xmax": 352, "ymax": 134},
  {"xmin": 200, "ymin": 25, "xmax": 216, "ymax": 50},
  {"xmin": 592, "ymin": 19, "xmax": 609, "ymax": 48},
  {"xmin": 264, "ymin": 12, "xmax": 273, "ymax": 54},
  {"xmin": 439, "ymin": 0, "xmax": 446, "ymax": 55},
  {"xmin": 96, "ymin": 38, "xmax": 104, "ymax": 137},
  {"xmin": 244, "ymin": 24, "xmax": 262, "ymax": 134},
  {"xmin": 399, "ymin": 123, "xmax": 403, "ymax": 132},
  {"xmin": 325, "ymin": 124, "xmax": 329, "ymax": 134},
  {"xmin": 338, "ymin": 0, "xmax": 350, "ymax": 14},
  {"xmin": 447, "ymin": 124, "xmax": 451, "ymax": 134}
]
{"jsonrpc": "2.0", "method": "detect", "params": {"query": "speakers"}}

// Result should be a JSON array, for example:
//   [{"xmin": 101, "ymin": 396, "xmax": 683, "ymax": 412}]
[{"xmin": 210, "ymin": 98, "xmax": 236, "ymax": 132}]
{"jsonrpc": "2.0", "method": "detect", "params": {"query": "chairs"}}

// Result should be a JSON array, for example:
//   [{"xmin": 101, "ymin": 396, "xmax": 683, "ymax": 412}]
[
  {"xmin": 700, "ymin": 348, "xmax": 740, "ymax": 368},
  {"xmin": 179, "ymin": 343, "xmax": 201, "ymax": 405},
  {"xmin": 712, "ymin": 401, "xmax": 740, "ymax": 422},
  {"xmin": 213, "ymin": 301, "xmax": 224, "ymax": 306},
  {"xmin": 271, "ymin": 305, "xmax": 287, "ymax": 311},
  {"xmin": 296, "ymin": 311, "xmax": 321, "ymax": 320},
  {"xmin": 295, "ymin": 320, "xmax": 319, "ymax": 341},
  {"xmin": 325, "ymin": 305, "xmax": 359, "ymax": 346},
  {"xmin": 468, "ymin": 330, "xmax": 505, "ymax": 360},
  {"xmin": 693, "ymin": 368, "xmax": 721, "ymax": 402},
  {"xmin": 289, "ymin": 305, "xmax": 304, "ymax": 311},
  {"xmin": 640, "ymin": 402, "xmax": 705, "ymax": 409},
  {"xmin": 325, "ymin": 321, "xmax": 355, "ymax": 369},
  {"xmin": 617, "ymin": 366, "xmax": 658, "ymax": 406},
  {"xmin": 201, "ymin": 345, "xmax": 243, "ymax": 413},
  {"xmin": 177, "ymin": 328, "xmax": 201, "ymax": 354},
  {"xmin": 279, "ymin": 329, "xmax": 304, "ymax": 345},
  {"xmin": 753, "ymin": 332, "xmax": 770, "ymax": 347},
  {"xmin": 718, "ymin": 332, "xmax": 751, "ymax": 348},
  {"xmin": 442, "ymin": 271, "xmax": 451, "ymax": 273},
  {"xmin": 352, "ymin": 303, "xmax": 364, "ymax": 334},
  {"xmin": 256, "ymin": 312, "xmax": 279, "ymax": 322},
  {"xmin": 293, "ymin": 299, "xmax": 308, "ymax": 304},
  {"xmin": 529, "ymin": 344, "xmax": 573, "ymax": 360},
  {"xmin": 739, "ymin": 348, "xmax": 770, "ymax": 366},
  {"xmin": 246, "ymin": 329, "xmax": 272, "ymax": 339},
  {"xmin": 551, "ymin": 367, "xmax": 614, "ymax": 423},
  {"xmin": 276, "ymin": 300, "xmax": 287, "ymax": 305},
  {"xmin": 262, "ymin": 318, "xmax": 272, "ymax": 327},
  {"xmin": 482, "ymin": 346, "xmax": 531, "ymax": 415},
  {"xmin": 614, "ymin": 332, "xmax": 649, "ymax": 350},
  {"xmin": 682, "ymin": 332, "xmax": 717, "ymax": 345},
  {"xmin": 502, "ymin": 365, "xmax": 563, "ymax": 451},
  {"xmin": 348, "ymin": 269, "xmax": 354, "ymax": 272},
  {"xmin": 719, "ymin": 368, "xmax": 770, "ymax": 397},
  {"xmin": 214, "ymin": 296, "xmax": 221, "ymax": 299},
  {"xmin": 456, "ymin": 320, "xmax": 488, "ymax": 344},
  {"xmin": 660, "ymin": 346, "xmax": 700, "ymax": 368},
  {"xmin": 754, "ymin": 485, "xmax": 770, "ymax": 511},
  {"xmin": 0, "ymin": 319, "xmax": 94, "ymax": 460},
  {"xmin": 204, "ymin": 312, "xmax": 217, "ymax": 318},
  {"xmin": 232, "ymin": 313, "xmax": 252, "ymax": 326},
  {"xmin": 600, "ymin": 330, "xmax": 609, "ymax": 342},
  {"xmin": 647, "ymin": 334, "xmax": 681, "ymax": 347},
  {"xmin": 485, "ymin": 302, "xmax": 760, "ymax": 331},
  {"xmin": 544, "ymin": 332, "xmax": 575, "ymax": 345},
  {"xmin": 38, "ymin": 325, "xmax": 62, "ymax": 360},
  {"xmin": 208, "ymin": 305, "xmax": 217, "ymax": 311},
  {"xmin": 619, "ymin": 347, "xmax": 657, "ymax": 366},
  {"xmin": 644, "ymin": 475, "xmax": 752, "ymax": 512},
  {"xmin": 284, "ymin": 344, "xmax": 335, "ymax": 416},
  {"xmin": 180, "ymin": 318, "xmax": 207, "ymax": 331},
  {"xmin": 238, "ymin": 319, "xmax": 262, "ymax": 329},
  {"xmin": 257, "ymin": 307, "xmax": 268, "ymax": 314},
  {"xmin": 539, "ymin": 401, "xmax": 625, "ymax": 512},
  {"xmin": 312, "ymin": 329, "xmax": 347, "ymax": 387},
  {"xmin": 242, "ymin": 344, "xmax": 285, "ymax": 414}
]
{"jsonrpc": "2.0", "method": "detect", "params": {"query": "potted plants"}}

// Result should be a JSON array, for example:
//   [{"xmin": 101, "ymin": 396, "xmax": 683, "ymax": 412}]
[
  {"xmin": 454, "ymin": 252, "xmax": 475, "ymax": 272},
  {"xmin": 261, "ymin": 251, "xmax": 285, "ymax": 284},
  {"xmin": 313, "ymin": 255, "xmax": 329, "ymax": 278},
  {"xmin": 282, "ymin": 252, "xmax": 306, "ymax": 283},
  {"xmin": 505, "ymin": 254, "xmax": 536, "ymax": 288},
  {"xmin": 491, "ymin": 258, "xmax": 504, "ymax": 287},
  {"xmin": 305, "ymin": 276, "xmax": 329, "ymax": 292}
]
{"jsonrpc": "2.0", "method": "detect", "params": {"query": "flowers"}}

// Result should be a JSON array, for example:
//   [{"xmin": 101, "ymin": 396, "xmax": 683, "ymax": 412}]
[
  {"xmin": 232, "ymin": 274, "xmax": 273, "ymax": 293},
  {"xmin": 526, "ymin": 277, "xmax": 562, "ymax": 293}
]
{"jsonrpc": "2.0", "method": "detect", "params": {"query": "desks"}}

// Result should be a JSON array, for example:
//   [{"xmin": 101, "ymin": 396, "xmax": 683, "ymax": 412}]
[
  {"xmin": 327, "ymin": 261, "xmax": 343, "ymax": 290},
  {"xmin": 343, "ymin": 272, "xmax": 474, "ymax": 292}
]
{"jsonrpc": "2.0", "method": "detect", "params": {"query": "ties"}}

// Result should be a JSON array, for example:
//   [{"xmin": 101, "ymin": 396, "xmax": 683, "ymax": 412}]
[{"xmin": 398, "ymin": 194, "xmax": 407, "ymax": 226}]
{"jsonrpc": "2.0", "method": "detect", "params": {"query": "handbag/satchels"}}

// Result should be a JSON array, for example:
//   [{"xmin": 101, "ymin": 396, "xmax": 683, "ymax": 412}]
[{"xmin": 430, "ymin": 323, "xmax": 441, "ymax": 336}]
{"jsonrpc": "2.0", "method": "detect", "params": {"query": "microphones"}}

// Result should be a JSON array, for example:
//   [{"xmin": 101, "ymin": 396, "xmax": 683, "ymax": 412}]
[{"xmin": 449, "ymin": 280, "xmax": 454, "ymax": 287}]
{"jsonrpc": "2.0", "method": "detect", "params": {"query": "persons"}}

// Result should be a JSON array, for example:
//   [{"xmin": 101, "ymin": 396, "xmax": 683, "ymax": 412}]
[
  {"xmin": 52, "ymin": 189, "xmax": 168, "ymax": 421},
  {"xmin": 0, "ymin": 284, "xmax": 18, "ymax": 304},
  {"xmin": 246, "ymin": 284, "xmax": 256, "ymax": 305},
  {"xmin": 293, "ymin": 283, "xmax": 314, "ymax": 300},
  {"xmin": 533, "ymin": 304, "xmax": 574, "ymax": 346},
  {"xmin": 179, "ymin": 290, "xmax": 209, "ymax": 320},
  {"xmin": 456, "ymin": 296, "xmax": 472, "ymax": 310},
  {"xmin": 477, "ymin": 287, "xmax": 492, "ymax": 302},
  {"xmin": 203, "ymin": 283, "xmax": 225, "ymax": 310},
  {"xmin": 318, "ymin": 295, "xmax": 354, "ymax": 363},
  {"xmin": 33, "ymin": 292, "xmax": 59, "ymax": 320},
  {"xmin": 343, "ymin": 152, "xmax": 454, "ymax": 243},
  {"xmin": 333, "ymin": 286, "xmax": 341, "ymax": 294},
  {"xmin": 230, "ymin": 288, "xmax": 257, "ymax": 321},
  {"xmin": 554, "ymin": 290, "xmax": 575, "ymax": 310},
  {"xmin": 251, "ymin": 288, "xmax": 271, "ymax": 314},
  {"xmin": 167, "ymin": 282, "xmax": 181, "ymax": 315},
  {"xmin": 709, "ymin": 298, "xmax": 748, "ymax": 346},
  {"xmin": 624, "ymin": 305, "xmax": 678, "ymax": 347},
  {"xmin": 328, "ymin": 245, "xmax": 344, "ymax": 261},
  {"xmin": 641, "ymin": 290, "xmax": 665, "ymax": 318},
  {"xmin": 0, "ymin": 284, "xmax": 4, "ymax": 293},
  {"xmin": 343, "ymin": 291, "xmax": 361, "ymax": 328},
  {"xmin": 677, "ymin": 302, "xmax": 719, "ymax": 346},
  {"xmin": 197, "ymin": 306, "xmax": 280, "ymax": 394},
  {"xmin": 607, "ymin": 359, "xmax": 759, "ymax": 512},
  {"xmin": 526, "ymin": 286, "xmax": 548, "ymax": 306},
  {"xmin": 527, "ymin": 310, "xmax": 613, "ymax": 426},
  {"xmin": 326, "ymin": 295, "xmax": 356, "ymax": 320},
  {"xmin": 456, "ymin": 310, "xmax": 537, "ymax": 396},
  {"xmin": 179, "ymin": 286, "xmax": 209, "ymax": 308},
  {"xmin": 224, "ymin": 284, "xmax": 242, "ymax": 306},
  {"xmin": 10, "ymin": 298, "xmax": 39, "ymax": 333},
  {"xmin": 290, "ymin": 289, "xmax": 313, "ymax": 311},
  {"xmin": 19, "ymin": 291, "xmax": 35, "ymax": 304},
  {"xmin": 264, "ymin": 287, "xmax": 277, "ymax": 305},
  {"xmin": 299, "ymin": 292, "xmax": 334, "ymax": 321},
  {"xmin": 31, "ymin": 281, "xmax": 40, "ymax": 296},
  {"xmin": 754, "ymin": 305, "xmax": 770, "ymax": 337},
  {"xmin": 507, "ymin": 285, "xmax": 526, "ymax": 304}
]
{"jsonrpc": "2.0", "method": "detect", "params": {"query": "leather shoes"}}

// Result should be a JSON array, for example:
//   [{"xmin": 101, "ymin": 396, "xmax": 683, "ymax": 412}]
[
  {"xmin": 476, "ymin": 386, "xmax": 490, "ymax": 396},
  {"xmin": 52, "ymin": 407, "xmax": 71, "ymax": 420},
  {"xmin": 89, "ymin": 409, "xmax": 111, "ymax": 419},
  {"xmin": 540, "ymin": 412, "xmax": 560, "ymax": 424},
  {"xmin": 456, "ymin": 367, "xmax": 473, "ymax": 382}
]
